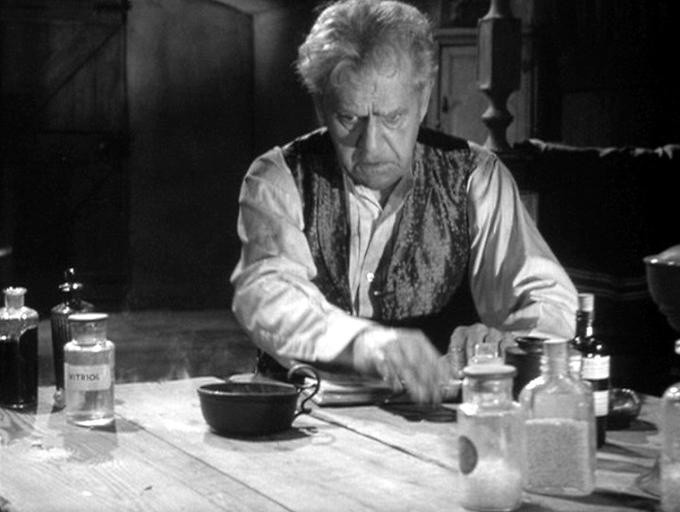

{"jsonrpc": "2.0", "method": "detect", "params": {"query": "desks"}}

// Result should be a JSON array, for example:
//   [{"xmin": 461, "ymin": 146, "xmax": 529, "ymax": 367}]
[{"xmin": 0, "ymin": 371, "xmax": 680, "ymax": 512}]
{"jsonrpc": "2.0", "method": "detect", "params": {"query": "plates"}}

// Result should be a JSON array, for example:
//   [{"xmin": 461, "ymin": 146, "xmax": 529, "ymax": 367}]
[{"xmin": 610, "ymin": 388, "xmax": 641, "ymax": 429}]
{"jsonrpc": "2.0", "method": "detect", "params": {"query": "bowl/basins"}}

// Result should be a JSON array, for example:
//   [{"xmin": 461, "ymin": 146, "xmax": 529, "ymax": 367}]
[{"xmin": 198, "ymin": 363, "xmax": 321, "ymax": 435}]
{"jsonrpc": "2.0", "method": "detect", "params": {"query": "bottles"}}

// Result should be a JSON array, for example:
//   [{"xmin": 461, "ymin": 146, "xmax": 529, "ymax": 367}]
[
  {"xmin": 0, "ymin": 267, "xmax": 115, "ymax": 428},
  {"xmin": 658, "ymin": 340, "xmax": 680, "ymax": 510},
  {"xmin": 457, "ymin": 291, "xmax": 611, "ymax": 511}
]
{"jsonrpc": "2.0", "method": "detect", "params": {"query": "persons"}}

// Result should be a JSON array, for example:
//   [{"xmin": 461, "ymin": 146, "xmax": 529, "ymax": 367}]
[{"xmin": 228, "ymin": 0, "xmax": 582, "ymax": 410}]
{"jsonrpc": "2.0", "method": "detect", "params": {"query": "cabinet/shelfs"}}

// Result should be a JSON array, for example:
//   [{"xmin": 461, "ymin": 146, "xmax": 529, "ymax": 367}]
[{"xmin": 423, "ymin": 26, "xmax": 542, "ymax": 230}]
